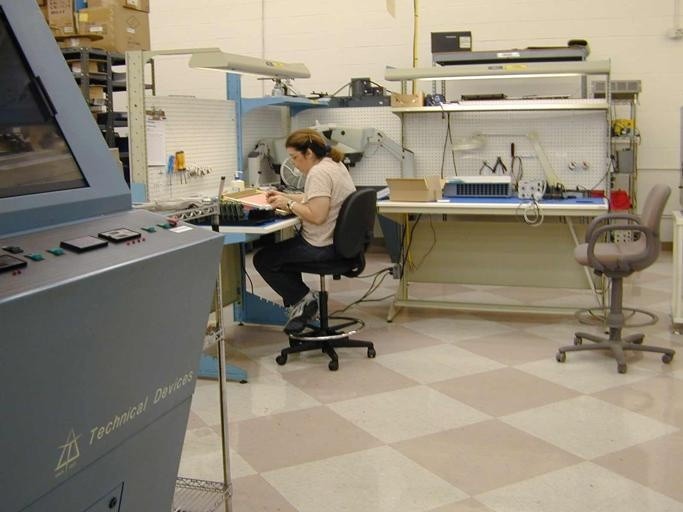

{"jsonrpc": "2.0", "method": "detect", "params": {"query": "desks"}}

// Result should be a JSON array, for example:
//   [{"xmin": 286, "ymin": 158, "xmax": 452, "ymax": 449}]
[
  {"xmin": 379, "ymin": 195, "xmax": 610, "ymax": 323},
  {"xmin": 188, "ymin": 192, "xmax": 320, "ymax": 384}
]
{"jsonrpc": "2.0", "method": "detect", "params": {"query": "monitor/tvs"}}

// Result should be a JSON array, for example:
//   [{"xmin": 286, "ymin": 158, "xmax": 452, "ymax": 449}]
[{"xmin": 1, "ymin": 1, "xmax": 90, "ymax": 202}]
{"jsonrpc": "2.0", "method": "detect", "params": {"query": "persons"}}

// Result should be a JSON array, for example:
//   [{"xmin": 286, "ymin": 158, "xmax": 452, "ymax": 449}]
[{"xmin": 253, "ymin": 128, "xmax": 357, "ymax": 335}]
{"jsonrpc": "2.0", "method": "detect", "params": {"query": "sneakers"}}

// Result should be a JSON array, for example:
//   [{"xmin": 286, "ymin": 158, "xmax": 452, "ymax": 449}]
[{"xmin": 282, "ymin": 289, "xmax": 320, "ymax": 337}]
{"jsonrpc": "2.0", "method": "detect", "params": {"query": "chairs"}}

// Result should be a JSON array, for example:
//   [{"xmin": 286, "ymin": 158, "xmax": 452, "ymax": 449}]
[
  {"xmin": 555, "ymin": 182, "xmax": 676, "ymax": 375},
  {"xmin": 274, "ymin": 187, "xmax": 377, "ymax": 371}
]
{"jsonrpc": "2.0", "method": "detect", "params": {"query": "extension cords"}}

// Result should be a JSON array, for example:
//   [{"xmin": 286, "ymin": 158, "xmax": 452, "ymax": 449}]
[{"xmin": 392, "ymin": 265, "xmax": 401, "ymax": 279}]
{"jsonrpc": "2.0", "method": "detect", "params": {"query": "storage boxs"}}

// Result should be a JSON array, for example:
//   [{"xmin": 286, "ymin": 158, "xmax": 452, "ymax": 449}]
[{"xmin": 38, "ymin": 0, "xmax": 150, "ymax": 113}]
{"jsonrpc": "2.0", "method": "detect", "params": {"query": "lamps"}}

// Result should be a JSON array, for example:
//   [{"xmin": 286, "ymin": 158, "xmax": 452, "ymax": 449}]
[
  {"xmin": 149, "ymin": 45, "xmax": 311, "ymax": 81},
  {"xmin": 383, "ymin": 57, "xmax": 612, "ymax": 83}
]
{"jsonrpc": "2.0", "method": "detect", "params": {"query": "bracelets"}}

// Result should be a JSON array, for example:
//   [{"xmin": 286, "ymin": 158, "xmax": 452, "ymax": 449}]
[
  {"xmin": 286, "ymin": 200, "xmax": 297, "ymax": 214},
  {"xmin": 289, "ymin": 193, "xmax": 291, "ymax": 198}
]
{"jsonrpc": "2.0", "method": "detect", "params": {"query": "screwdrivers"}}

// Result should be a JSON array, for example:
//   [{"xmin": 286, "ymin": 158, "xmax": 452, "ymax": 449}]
[{"xmin": 168, "ymin": 151, "xmax": 187, "ymax": 185}]
